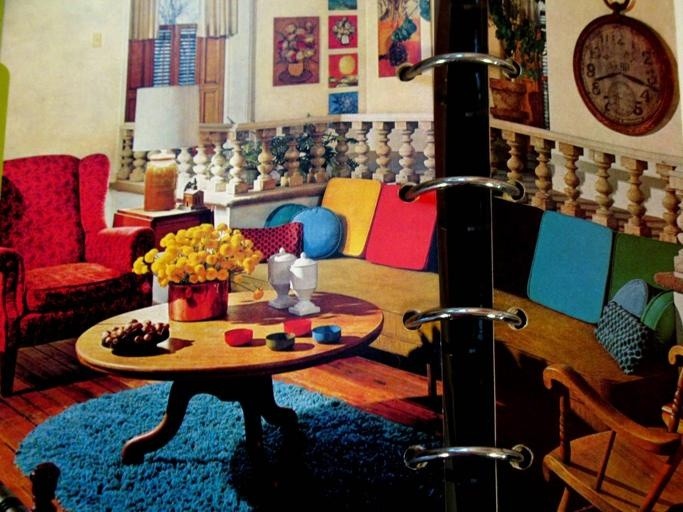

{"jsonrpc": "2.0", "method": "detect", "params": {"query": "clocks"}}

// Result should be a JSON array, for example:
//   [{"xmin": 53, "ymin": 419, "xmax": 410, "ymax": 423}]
[{"xmin": 572, "ymin": 3, "xmax": 674, "ymax": 137}]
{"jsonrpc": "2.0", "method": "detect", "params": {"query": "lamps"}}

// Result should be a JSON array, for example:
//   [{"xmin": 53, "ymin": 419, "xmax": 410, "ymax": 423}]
[{"xmin": 132, "ymin": 81, "xmax": 203, "ymax": 214}]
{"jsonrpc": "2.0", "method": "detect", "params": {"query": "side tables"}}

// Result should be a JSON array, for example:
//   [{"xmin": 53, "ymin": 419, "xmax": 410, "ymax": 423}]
[{"xmin": 111, "ymin": 206, "xmax": 213, "ymax": 254}]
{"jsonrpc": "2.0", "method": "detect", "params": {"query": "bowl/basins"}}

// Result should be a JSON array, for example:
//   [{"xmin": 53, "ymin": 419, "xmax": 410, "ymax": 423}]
[{"xmin": 225, "ymin": 318, "xmax": 341, "ymax": 351}]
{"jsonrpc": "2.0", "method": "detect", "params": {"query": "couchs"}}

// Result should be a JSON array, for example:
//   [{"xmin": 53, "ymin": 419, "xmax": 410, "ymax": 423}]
[{"xmin": 233, "ymin": 177, "xmax": 683, "ymax": 434}]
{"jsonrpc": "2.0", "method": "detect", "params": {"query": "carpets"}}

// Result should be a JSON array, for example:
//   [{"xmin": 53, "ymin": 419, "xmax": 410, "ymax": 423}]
[{"xmin": 14, "ymin": 376, "xmax": 451, "ymax": 512}]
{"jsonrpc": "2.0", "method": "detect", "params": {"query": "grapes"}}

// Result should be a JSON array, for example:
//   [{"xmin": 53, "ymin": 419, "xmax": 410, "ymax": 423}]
[{"xmin": 105, "ymin": 319, "xmax": 163, "ymax": 344}]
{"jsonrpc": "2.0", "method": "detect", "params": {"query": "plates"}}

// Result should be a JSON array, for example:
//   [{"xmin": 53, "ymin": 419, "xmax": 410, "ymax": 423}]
[{"xmin": 102, "ymin": 328, "xmax": 168, "ymax": 356}]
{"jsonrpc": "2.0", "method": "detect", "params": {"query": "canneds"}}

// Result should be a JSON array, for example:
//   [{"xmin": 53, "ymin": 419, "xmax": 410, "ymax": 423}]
[{"xmin": 144, "ymin": 152, "xmax": 178, "ymax": 211}]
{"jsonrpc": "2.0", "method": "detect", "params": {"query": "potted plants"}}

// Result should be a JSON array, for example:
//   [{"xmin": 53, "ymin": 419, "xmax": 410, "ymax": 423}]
[{"xmin": 486, "ymin": 0, "xmax": 550, "ymax": 125}]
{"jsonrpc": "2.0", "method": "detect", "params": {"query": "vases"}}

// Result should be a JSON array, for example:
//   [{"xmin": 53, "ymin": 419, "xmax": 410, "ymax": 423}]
[{"xmin": 166, "ymin": 283, "xmax": 230, "ymax": 321}]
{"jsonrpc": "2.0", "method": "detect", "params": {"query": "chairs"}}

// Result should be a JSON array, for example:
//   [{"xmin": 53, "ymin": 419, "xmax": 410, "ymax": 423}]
[
  {"xmin": 539, "ymin": 344, "xmax": 683, "ymax": 512},
  {"xmin": 0, "ymin": 152, "xmax": 160, "ymax": 398}
]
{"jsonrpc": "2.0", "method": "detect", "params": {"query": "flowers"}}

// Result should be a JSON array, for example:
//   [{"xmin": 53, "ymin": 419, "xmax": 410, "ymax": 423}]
[{"xmin": 132, "ymin": 222, "xmax": 264, "ymax": 291}]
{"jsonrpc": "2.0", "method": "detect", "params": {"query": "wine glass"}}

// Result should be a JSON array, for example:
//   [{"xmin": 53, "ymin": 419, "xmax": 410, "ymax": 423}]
[{"xmin": 267, "ymin": 248, "xmax": 321, "ymax": 316}]
